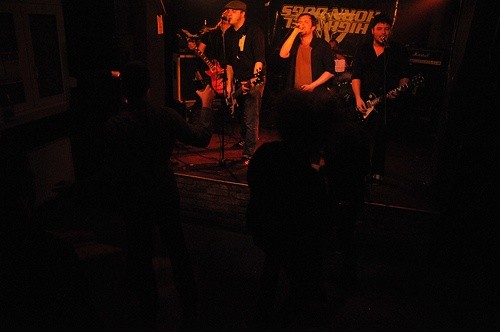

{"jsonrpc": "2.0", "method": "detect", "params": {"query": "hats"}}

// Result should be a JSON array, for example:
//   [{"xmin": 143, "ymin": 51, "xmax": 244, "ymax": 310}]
[{"xmin": 225, "ymin": 1, "xmax": 247, "ymax": 11}]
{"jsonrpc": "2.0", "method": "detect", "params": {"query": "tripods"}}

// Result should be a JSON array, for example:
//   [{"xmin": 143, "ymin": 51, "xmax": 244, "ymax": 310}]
[{"xmin": 184, "ymin": 20, "xmax": 248, "ymax": 184}]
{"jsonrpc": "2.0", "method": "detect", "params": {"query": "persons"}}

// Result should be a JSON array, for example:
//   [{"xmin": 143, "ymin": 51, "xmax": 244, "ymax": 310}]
[
  {"xmin": 0, "ymin": 138, "xmax": 95, "ymax": 332},
  {"xmin": 249, "ymin": 92, "xmax": 371, "ymax": 332},
  {"xmin": 279, "ymin": 14, "xmax": 335, "ymax": 91},
  {"xmin": 351, "ymin": 14, "xmax": 412, "ymax": 184},
  {"xmin": 183, "ymin": 0, "xmax": 267, "ymax": 159},
  {"xmin": 107, "ymin": 61, "xmax": 217, "ymax": 332}
]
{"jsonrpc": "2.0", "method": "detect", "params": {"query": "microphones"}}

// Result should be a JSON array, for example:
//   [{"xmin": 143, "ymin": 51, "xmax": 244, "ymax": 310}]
[
  {"xmin": 291, "ymin": 23, "xmax": 299, "ymax": 28},
  {"xmin": 382, "ymin": 37, "xmax": 389, "ymax": 48},
  {"xmin": 221, "ymin": 15, "xmax": 230, "ymax": 22}
]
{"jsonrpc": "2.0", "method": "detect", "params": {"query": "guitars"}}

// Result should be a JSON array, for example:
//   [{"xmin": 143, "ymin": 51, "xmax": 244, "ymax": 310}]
[
  {"xmin": 352, "ymin": 73, "xmax": 424, "ymax": 128},
  {"xmin": 225, "ymin": 70, "xmax": 265, "ymax": 120},
  {"xmin": 177, "ymin": 28, "xmax": 226, "ymax": 94}
]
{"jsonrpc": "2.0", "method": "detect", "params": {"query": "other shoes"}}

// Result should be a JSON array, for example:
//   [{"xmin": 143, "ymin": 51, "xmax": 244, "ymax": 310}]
[
  {"xmin": 232, "ymin": 156, "xmax": 250, "ymax": 169},
  {"xmin": 372, "ymin": 175, "xmax": 383, "ymax": 186}
]
